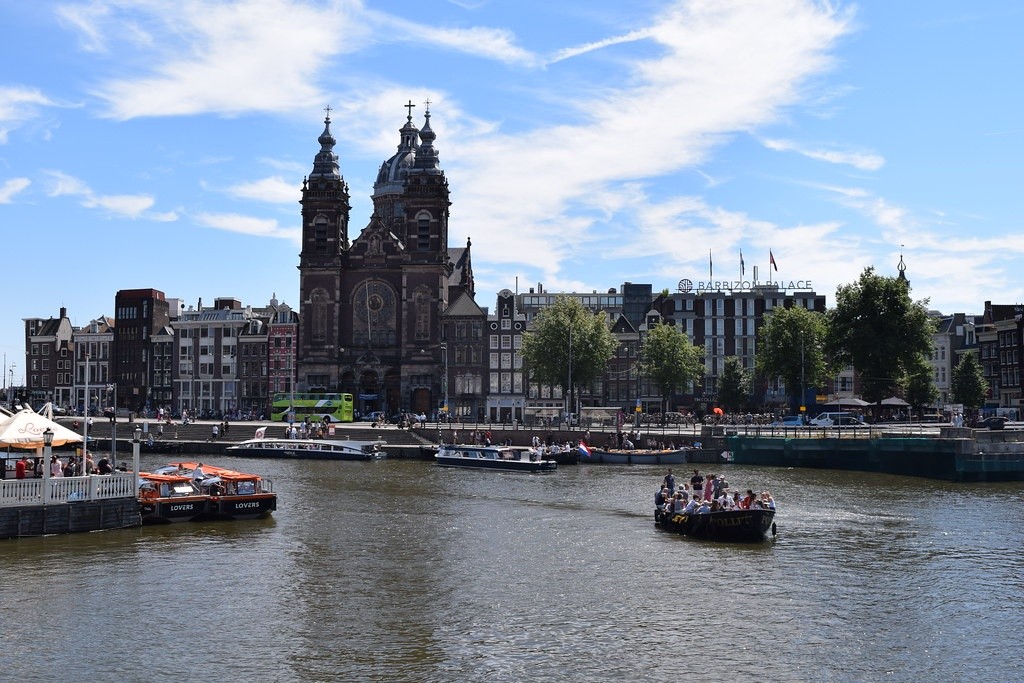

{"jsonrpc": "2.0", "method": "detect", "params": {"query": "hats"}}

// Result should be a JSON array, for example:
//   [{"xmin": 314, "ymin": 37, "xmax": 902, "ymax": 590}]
[{"xmin": 720, "ymin": 475, "xmax": 725, "ymax": 479}]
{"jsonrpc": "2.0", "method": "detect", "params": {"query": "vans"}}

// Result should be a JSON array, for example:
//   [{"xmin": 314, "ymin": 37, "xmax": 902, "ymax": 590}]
[{"xmin": 809, "ymin": 412, "xmax": 851, "ymax": 426}]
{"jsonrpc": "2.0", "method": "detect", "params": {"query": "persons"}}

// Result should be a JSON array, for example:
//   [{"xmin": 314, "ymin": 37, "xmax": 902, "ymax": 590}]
[
  {"xmin": 469, "ymin": 430, "xmax": 492, "ymax": 445},
  {"xmin": 939, "ymin": 408, "xmax": 984, "ymax": 427},
  {"xmin": 88, "ymin": 418, "xmax": 93, "ymax": 433},
  {"xmin": 15, "ymin": 456, "xmax": 28, "ymax": 478},
  {"xmin": 73, "ymin": 408, "xmax": 77, "ymax": 416},
  {"xmin": 655, "ymin": 469, "xmax": 775, "ymax": 515},
  {"xmin": 201, "ymin": 407, "xmax": 266, "ymax": 441},
  {"xmin": 602, "ymin": 430, "xmax": 703, "ymax": 453},
  {"xmin": 285, "ymin": 419, "xmax": 329, "ymax": 449},
  {"xmin": 167, "ymin": 463, "xmax": 183, "ymax": 492},
  {"xmin": 506, "ymin": 412, "xmax": 569, "ymax": 428},
  {"xmin": 37, "ymin": 451, "xmax": 113, "ymax": 478},
  {"xmin": 353, "ymin": 408, "xmax": 358, "ymax": 419},
  {"xmin": 193, "ymin": 463, "xmax": 255, "ymax": 494},
  {"xmin": 532, "ymin": 426, "xmax": 590, "ymax": 460},
  {"xmin": 376, "ymin": 411, "xmax": 390, "ymax": 428},
  {"xmin": 434, "ymin": 411, "xmax": 451, "ymax": 422},
  {"xmin": 143, "ymin": 405, "xmax": 197, "ymax": 445},
  {"xmin": 857, "ymin": 409, "xmax": 906, "ymax": 422},
  {"xmin": 501, "ymin": 437, "xmax": 512, "ymax": 445},
  {"xmin": 623, "ymin": 410, "xmax": 783, "ymax": 425},
  {"xmin": 73, "ymin": 421, "xmax": 80, "ymax": 432},
  {"xmin": 399, "ymin": 412, "xmax": 427, "ymax": 430}
]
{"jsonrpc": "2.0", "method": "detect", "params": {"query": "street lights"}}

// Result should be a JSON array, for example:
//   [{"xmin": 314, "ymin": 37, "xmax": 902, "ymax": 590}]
[
  {"xmin": 131, "ymin": 425, "xmax": 143, "ymax": 498},
  {"xmin": 623, "ymin": 346, "xmax": 642, "ymax": 425},
  {"xmin": 801, "ymin": 329, "xmax": 805, "ymax": 415},
  {"xmin": 567, "ymin": 343, "xmax": 593, "ymax": 425},
  {"xmin": 420, "ymin": 342, "xmax": 450, "ymax": 423},
  {"xmin": 42, "ymin": 428, "xmax": 54, "ymax": 504},
  {"xmin": 335, "ymin": 346, "xmax": 346, "ymax": 392}
]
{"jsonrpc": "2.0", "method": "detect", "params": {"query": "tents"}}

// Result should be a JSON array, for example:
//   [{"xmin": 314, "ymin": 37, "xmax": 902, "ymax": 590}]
[{"xmin": 0, "ymin": 409, "xmax": 84, "ymax": 465}]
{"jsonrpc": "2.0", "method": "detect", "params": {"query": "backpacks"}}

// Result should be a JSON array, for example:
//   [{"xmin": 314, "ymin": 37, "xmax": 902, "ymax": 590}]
[{"xmin": 64, "ymin": 464, "xmax": 74, "ymax": 477}]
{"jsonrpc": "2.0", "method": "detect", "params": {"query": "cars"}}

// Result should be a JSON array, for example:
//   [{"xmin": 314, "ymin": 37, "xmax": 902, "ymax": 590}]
[
  {"xmin": 976, "ymin": 417, "xmax": 1013, "ymax": 430},
  {"xmin": 360, "ymin": 412, "xmax": 384, "ymax": 422},
  {"xmin": 393, "ymin": 413, "xmax": 420, "ymax": 424},
  {"xmin": 35, "ymin": 404, "xmax": 66, "ymax": 415}
]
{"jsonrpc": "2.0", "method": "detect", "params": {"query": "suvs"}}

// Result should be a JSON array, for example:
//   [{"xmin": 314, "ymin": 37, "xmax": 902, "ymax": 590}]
[
  {"xmin": 770, "ymin": 416, "xmax": 803, "ymax": 426},
  {"xmin": 833, "ymin": 417, "xmax": 865, "ymax": 426}
]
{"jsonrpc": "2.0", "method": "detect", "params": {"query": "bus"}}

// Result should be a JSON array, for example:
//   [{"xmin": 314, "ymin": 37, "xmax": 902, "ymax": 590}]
[
  {"xmin": 912, "ymin": 414, "xmax": 946, "ymax": 424},
  {"xmin": 271, "ymin": 393, "xmax": 353, "ymax": 423}
]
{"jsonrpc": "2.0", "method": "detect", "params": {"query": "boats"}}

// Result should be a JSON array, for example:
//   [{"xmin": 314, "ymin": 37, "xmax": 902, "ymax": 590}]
[
  {"xmin": 654, "ymin": 492, "xmax": 777, "ymax": 540},
  {"xmin": 558, "ymin": 451, "xmax": 578, "ymax": 465},
  {"xmin": 432, "ymin": 443, "xmax": 557, "ymax": 472},
  {"xmin": 115, "ymin": 461, "xmax": 277, "ymax": 523},
  {"xmin": 578, "ymin": 448, "xmax": 688, "ymax": 464},
  {"xmin": 224, "ymin": 439, "xmax": 387, "ymax": 460}
]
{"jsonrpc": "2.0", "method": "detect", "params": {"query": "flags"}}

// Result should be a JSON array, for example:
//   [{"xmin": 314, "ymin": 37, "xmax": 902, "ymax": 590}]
[
  {"xmin": 740, "ymin": 255, "xmax": 745, "ymax": 275},
  {"xmin": 579, "ymin": 442, "xmax": 590, "ymax": 456},
  {"xmin": 770, "ymin": 251, "xmax": 777, "ymax": 271}
]
